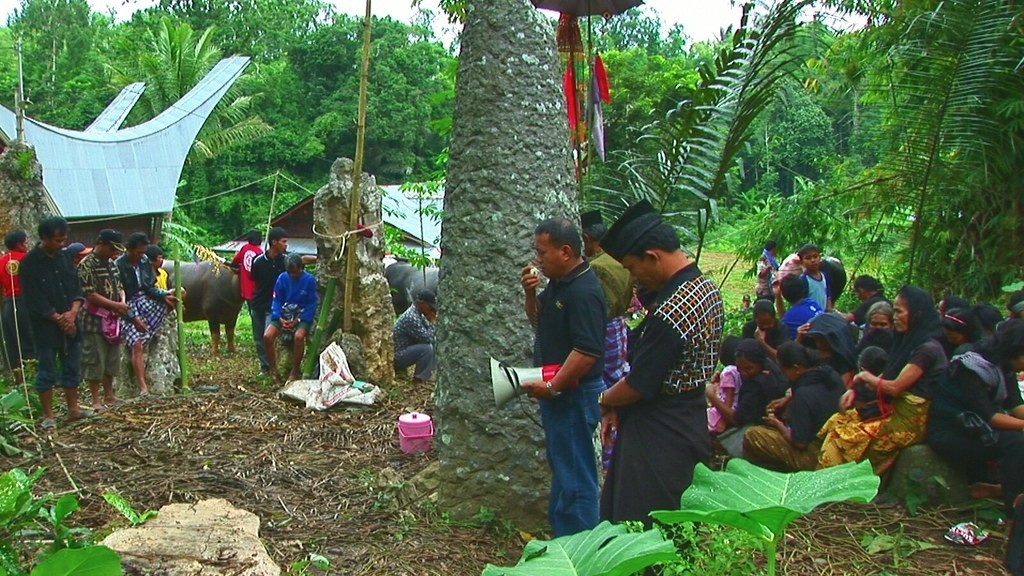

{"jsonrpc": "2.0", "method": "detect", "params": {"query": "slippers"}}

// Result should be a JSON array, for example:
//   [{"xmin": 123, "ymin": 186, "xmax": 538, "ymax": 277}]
[
  {"xmin": 41, "ymin": 420, "xmax": 57, "ymax": 431},
  {"xmin": 68, "ymin": 411, "xmax": 94, "ymax": 420}
]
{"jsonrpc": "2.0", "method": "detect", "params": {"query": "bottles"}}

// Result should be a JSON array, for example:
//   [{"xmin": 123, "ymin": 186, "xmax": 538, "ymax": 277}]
[{"xmin": 834, "ymin": 308, "xmax": 846, "ymax": 319}]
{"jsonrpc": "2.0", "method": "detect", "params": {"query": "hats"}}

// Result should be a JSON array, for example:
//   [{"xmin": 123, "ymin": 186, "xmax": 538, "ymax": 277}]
[
  {"xmin": 599, "ymin": 198, "xmax": 662, "ymax": 262},
  {"xmin": 99, "ymin": 229, "xmax": 127, "ymax": 252},
  {"xmin": 393, "ymin": 254, "xmax": 410, "ymax": 260},
  {"xmin": 581, "ymin": 210, "xmax": 602, "ymax": 228},
  {"xmin": 419, "ymin": 289, "xmax": 436, "ymax": 310},
  {"xmin": 67, "ymin": 243, "xmax": 93, "ymax": 254}
]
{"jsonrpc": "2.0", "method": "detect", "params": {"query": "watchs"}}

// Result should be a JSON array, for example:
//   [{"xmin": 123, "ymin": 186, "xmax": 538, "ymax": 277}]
[
  {"xmin": 545, "ymin": 380, "xmax": 565, "ymax": 400},
  {"xmin": 598, "ymin": 391, "xmax": 604, "ymax": 406}
]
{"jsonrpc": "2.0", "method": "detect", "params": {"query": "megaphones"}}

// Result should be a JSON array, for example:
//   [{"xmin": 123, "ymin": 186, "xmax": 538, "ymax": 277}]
[{"xmin": 489, "ymin": 356, "xmax": 579, "ymax": 410}]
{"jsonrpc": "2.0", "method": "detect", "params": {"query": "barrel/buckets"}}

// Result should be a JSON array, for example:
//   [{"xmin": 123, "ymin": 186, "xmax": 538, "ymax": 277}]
[{"xmin": 398, "ymin": 412, "xmax": 434, "ymax": 454}]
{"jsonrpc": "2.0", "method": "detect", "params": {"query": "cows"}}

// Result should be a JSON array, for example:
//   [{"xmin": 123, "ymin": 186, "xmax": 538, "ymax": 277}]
[{"xmin": 161, "ymin": 257, "xmax": 245, "ymax": 356}]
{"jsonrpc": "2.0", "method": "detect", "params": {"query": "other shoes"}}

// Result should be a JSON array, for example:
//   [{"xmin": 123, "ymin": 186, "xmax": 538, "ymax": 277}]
[
  {"xmin": 95, "ymin": 406, "xmax": 105, "ymax": 412},
  {"xmin": 257, "ymin": 371, "xmax": 269, "ymax": 376},
  {"xmin": 105, "ymin": 398, "xmax": 126, "ymax": 405},
  {"xmin": 412, "ymin": 377, "xmax": 422, "ymax": 387},
  {"xmin": 140, "ymin": 392, "xmax": 149, "ymax": 397}
]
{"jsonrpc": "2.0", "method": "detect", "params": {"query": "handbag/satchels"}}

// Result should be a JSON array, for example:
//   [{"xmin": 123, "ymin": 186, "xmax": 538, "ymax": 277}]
[{"xmin": 83, "ymin": 302, "xmax": 122, "ymax": 344}]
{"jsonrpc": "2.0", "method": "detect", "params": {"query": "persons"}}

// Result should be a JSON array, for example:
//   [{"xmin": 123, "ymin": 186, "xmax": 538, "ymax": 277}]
[
  {"xmin": 392, "ymin": 289, "xmax": 438, "ymax": 384},
  {"xmin": 232, "ymin": 227, "xmax": 320, "ymax": 391},
  {"xmin": 1, "ymin": 217, "xmax": 183, "ymax": 433},
  {"xmin": 582, "ymin": 210, "xmax": 644, "ymax": 475},
  {"xmin": 517, "ymin": 217, "xmax": 608, "ymax": 538},
  {"xmin": 599, "ymin": 200, "xmax": 725, "ymax": 576},
  {"xmin": 706, "ymin": 241, "xmax": 1024, "ymax": 576}
]
